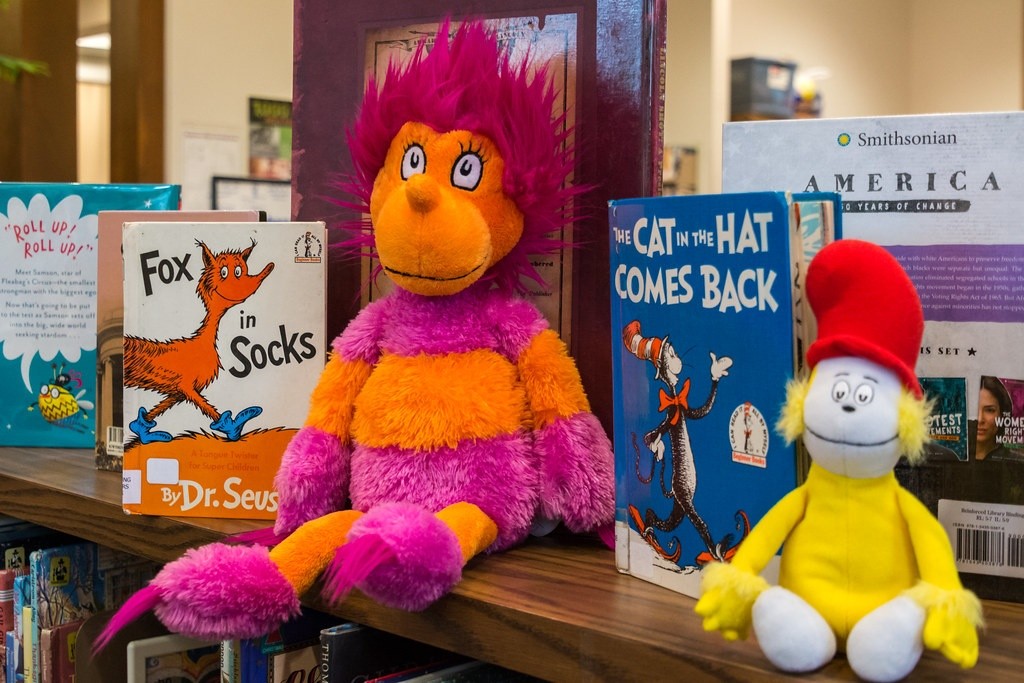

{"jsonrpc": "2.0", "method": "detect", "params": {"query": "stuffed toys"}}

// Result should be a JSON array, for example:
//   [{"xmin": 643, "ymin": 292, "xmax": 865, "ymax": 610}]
[
  {"xmin": 89, "ymin": 7, "xmax": 616, "ymax": 657},
  {"xmin": 695, "ymin": 239, "xmax": 984, "ymax": 682}
]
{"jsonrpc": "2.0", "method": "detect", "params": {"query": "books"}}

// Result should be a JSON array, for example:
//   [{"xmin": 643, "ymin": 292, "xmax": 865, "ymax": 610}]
[
  {"xmin": 607, "ymin": 193, "xmax": 1024, "ymax": 602},
  {"xmin": 0, "ymin": 180, "xmax": 531, "ymax": 683}
]
